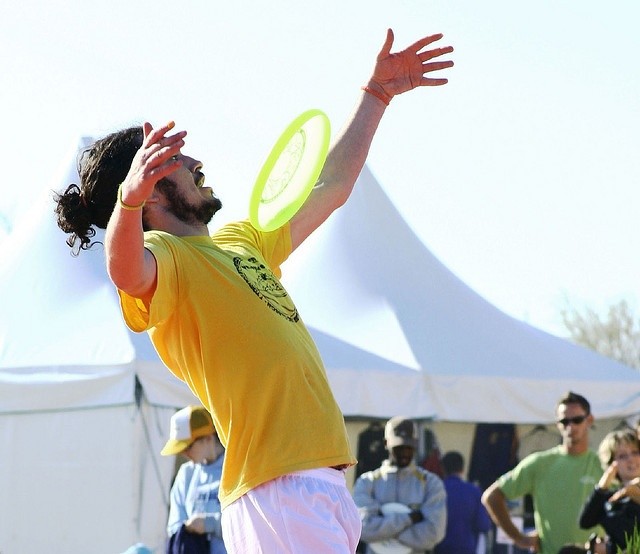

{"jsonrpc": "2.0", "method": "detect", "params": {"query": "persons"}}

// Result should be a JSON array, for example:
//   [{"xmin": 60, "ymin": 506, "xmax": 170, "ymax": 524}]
[
  {"xmin": 55, "ymin": 28, "xmax": 454, "ymax": 554},
  {"xmin": 580, "ymin": 429, "xmax": 640, "ymax": 553},
  {"xmin": 481, "ymin": 392, "xmax": 619, "ymax": 553},
  {"xmin": 352, "ymin": 415, "xmax": 448, "ymax": 554},
  {"xmin": 160, "ymin": 405, "xmax": 226, "ymax": 553},
  {"xmin": 435, "ymin": 451, "xmax": 491, "ymax": 554}
]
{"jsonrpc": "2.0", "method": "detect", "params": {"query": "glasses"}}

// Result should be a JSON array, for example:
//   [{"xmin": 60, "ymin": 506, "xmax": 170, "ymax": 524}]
[{"xmin": 557, "ymin": 415, "xmax": 588, "ymax": 425}]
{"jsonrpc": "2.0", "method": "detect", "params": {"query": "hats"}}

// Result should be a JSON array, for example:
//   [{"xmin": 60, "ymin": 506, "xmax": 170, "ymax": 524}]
[
  {"xmin": 161, "ymin": 405, "xmax": 214, "ymax": 456},
  {"xmin": 385, "ymin": 416, "xmax": 418, "ymax": 449}
]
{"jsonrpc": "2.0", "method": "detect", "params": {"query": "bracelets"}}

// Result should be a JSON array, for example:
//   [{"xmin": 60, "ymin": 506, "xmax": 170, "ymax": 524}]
[
  {"xmin": 117, "ymin": 182, "xmax": 146, "ymax": 211},
  {"xmin": 361, "ymin": 85, "xmax": 389, "ymax": 106}
]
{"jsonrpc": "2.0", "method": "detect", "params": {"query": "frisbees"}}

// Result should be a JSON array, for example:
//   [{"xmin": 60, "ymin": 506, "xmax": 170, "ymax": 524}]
[{"xmin": 249, "ymin": 108, "xmax": 332, "ymax": 233}]
